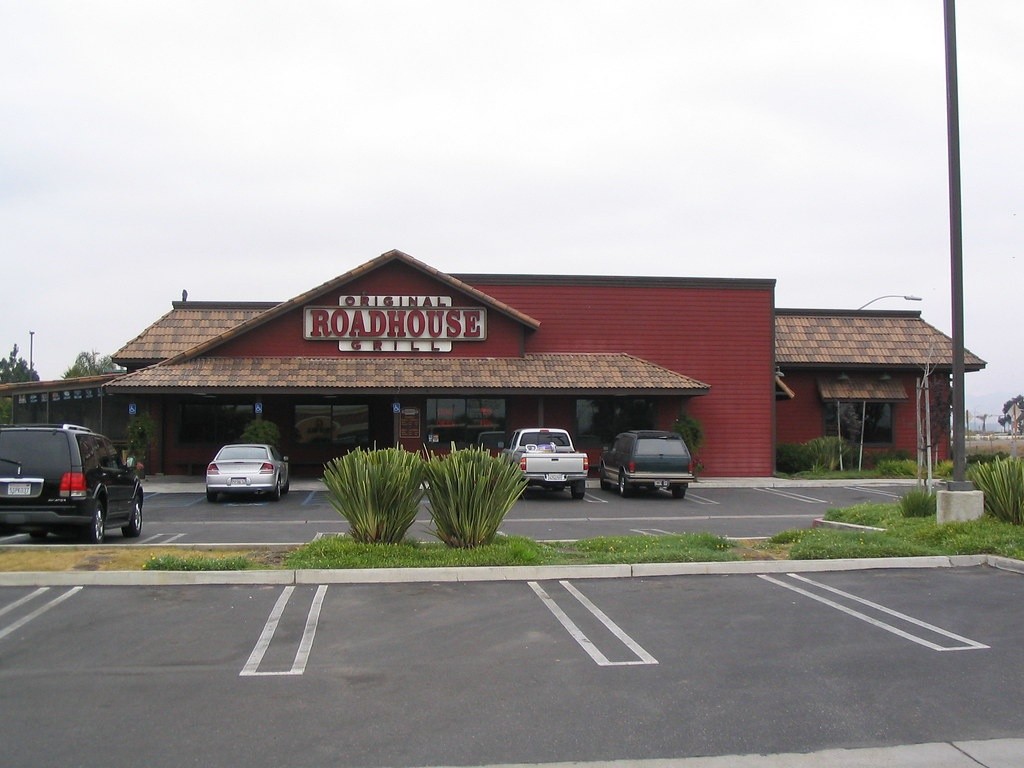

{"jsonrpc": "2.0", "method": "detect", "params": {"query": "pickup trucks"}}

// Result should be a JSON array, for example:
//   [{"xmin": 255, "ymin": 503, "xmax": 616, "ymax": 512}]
[{"xmin": 500, "ymin": 428, "xmax": 589, "ymax": 500}]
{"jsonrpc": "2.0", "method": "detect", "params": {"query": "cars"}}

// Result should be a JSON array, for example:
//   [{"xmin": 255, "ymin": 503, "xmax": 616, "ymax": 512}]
[{"xmin": 206, "ymin": 443, "xmax": 291, "ymax": 501}]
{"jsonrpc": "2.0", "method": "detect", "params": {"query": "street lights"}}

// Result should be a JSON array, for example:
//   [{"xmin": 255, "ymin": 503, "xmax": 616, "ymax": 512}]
[{"xmin": 29, "ymin": 330, "xmax": 36, "ymax": 382}]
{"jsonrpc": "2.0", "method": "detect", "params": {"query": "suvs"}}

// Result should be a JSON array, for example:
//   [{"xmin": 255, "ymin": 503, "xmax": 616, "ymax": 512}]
[
  {"xmin": 596, "ymin": 430, "xmax": 695, "ymax": 498},
  {"xmin": 0, "ymin": 423, "xmax": 143, "ymax": 545}
]
